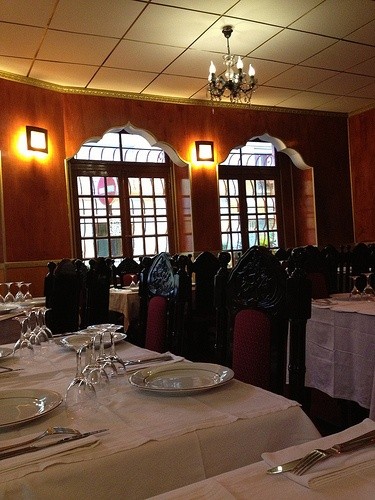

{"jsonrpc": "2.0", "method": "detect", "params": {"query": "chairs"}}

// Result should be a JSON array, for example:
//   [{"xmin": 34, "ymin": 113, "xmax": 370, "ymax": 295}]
[{"xmin": 43, "ymin": 244, "xmax": 374, "ymax": 389}]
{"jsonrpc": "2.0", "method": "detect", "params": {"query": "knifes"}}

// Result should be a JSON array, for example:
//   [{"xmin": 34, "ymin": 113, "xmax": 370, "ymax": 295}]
[
  {"xmin": 267, "ymin": 429, "xmax": 375, "ymax": 475},
  {"xmin": 123, "ymin": 355, "xmax": 172, "ymax": 365},
  {"xmin": 0, "ymin": 429, "xmax": 109, "ymax": 460}
]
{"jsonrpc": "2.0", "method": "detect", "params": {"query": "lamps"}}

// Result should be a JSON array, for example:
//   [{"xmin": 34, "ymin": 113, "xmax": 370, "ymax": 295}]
[
  {"xmin": 195, "ymin": 141, "xmax": 214, "ymax": 161},
  {"xmin": 25, "ymin": 126, "xmax": 50, "ymax": 153},
  {"xmin": 207, "ymin": 25, "xmax": 257, "ymax": 103}
]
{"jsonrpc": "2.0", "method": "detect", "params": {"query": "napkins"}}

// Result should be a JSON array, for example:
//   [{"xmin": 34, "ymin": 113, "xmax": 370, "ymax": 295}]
[
  {"xmin": 261, "ymin": 418, "xmax": 375, "ymax": 488},
  {"xmin": 0, "ymin": 424, "xmax": 101, "ymax": 472},
  {"xmin": 106, "ymin": 351, "xmax": 184, "ymax": 377}
]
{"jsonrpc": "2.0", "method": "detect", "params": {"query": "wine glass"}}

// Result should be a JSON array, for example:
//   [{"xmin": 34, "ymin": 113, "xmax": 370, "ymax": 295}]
[
  {"xmin": 0, "ymin": 281, "xmax": 53, "ymax": 359},
  {"xmin": 60, "ymin": 323, "xmax": 127, "ymax": 419},
  {"xmin": 361, "ymin": 272, "xmax": 375, "ymax": 304},
  {"xmin": 137, "ymin": 273, "xmax": 140, "ymax": 286},
  {"xmin": 130, "ymin": 275, "xmax": 136, "ymax": 287},
  {"xmin": 349, "ymin": 276, "xmax": 361, "ymax": 302}
]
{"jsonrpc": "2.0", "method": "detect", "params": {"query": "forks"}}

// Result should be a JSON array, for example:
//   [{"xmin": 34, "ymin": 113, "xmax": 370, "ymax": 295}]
[
  {"xmin": 290, "ymin": 435, "xmax": 375, "ymax": 476},
  {"xmin": 0, "ymin": 426, "xmax": 80, "ymax": 452}
]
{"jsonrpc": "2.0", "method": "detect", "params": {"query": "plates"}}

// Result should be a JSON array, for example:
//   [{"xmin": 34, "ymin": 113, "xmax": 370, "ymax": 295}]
[
  {"xmin": 0, "ymin": 347, "xmax": 13, "ymax": 359},
  {"xmin": 127, "ymin": 362, "xmax": 235, "ymax": 396},
  {"xmin": 130, "ymin": 287, "xmax": 139, "ymax": 291},
  {"xmin": 60, "ymin": 332, "xmax": 126, "ymax": 350},
  {"xmin": 0, "ymin": 389, "xmax": 63, "ymax": 427},
  {"xmin": 329, "ymin": 293, "xmax": 370, "ymax": 301}
]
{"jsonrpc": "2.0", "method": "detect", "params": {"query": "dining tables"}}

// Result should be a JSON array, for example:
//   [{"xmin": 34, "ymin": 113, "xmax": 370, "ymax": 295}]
[
  {"xmin": 0, "ymin": 341, "xmax": 322, "ymax": 500},
  {"xmin": 0, "ymin": 296, "xmax": 46, "ymax": 344},
  {"xmin": 306, "ymin": 298, "xmax": 375, "ymax": 422},
  {"xmin": 148, "ymin": 461, "xmax": 375, "ymax": 500},
  {"xmin": 109, "ymin": 284, "xmax": 139, "ymax": 333}
]
